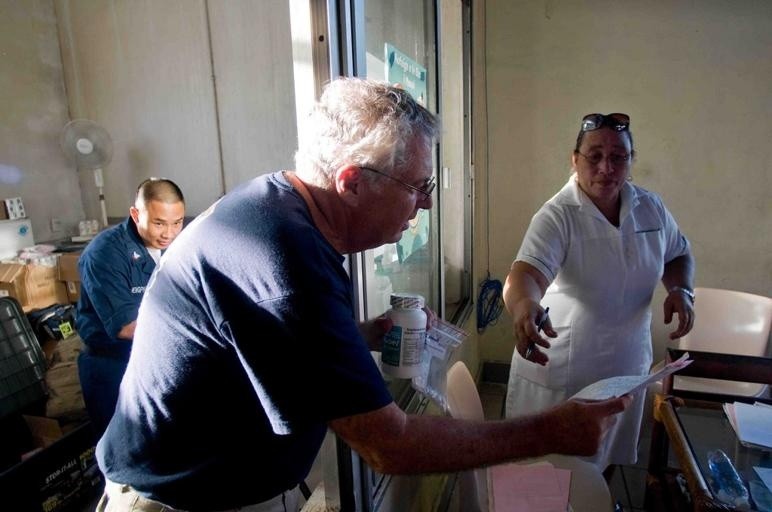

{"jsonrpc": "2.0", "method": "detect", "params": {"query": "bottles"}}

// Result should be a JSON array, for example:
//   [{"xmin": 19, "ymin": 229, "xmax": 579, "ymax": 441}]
[
  {"xmin": 706, "ymin": 448, "xmax": 753, "ymax": 512},
  {"xmin": 378, "ymin": 289, "xmax": 429, "ymax": 380}
]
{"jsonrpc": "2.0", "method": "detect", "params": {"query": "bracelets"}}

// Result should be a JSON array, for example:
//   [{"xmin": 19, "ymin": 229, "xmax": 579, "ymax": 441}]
[{"xmin": 667, "ymin": 285, "xmax": 698, "ymax": 305}]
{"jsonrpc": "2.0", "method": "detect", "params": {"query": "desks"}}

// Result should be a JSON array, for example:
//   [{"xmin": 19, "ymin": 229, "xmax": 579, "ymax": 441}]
[{"xmin": 644, "ymin": 391, "xmax": 772, "ymax": 512}]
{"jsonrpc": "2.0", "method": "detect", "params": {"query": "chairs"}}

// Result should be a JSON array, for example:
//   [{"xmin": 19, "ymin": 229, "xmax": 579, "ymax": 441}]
[
  {"xmin": 647, "ymin": 288, "xmax": 772, "ymax": 468},
  {"xmin": 447, "ymin": 361, "xmax": 614, "ymax": 512}
]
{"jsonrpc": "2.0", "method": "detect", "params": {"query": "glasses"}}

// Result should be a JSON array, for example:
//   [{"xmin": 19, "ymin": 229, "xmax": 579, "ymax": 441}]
[
  {"xmin": 576, "ymin": 148, "xmax": 634, "ymax": 169},
  {"xmin": 352, "ymin": 166, "xmax": 438, "ymax": 203},
  {"xmin": 580, "ymin": 113, "xmax": 632, "ymax": 133}
]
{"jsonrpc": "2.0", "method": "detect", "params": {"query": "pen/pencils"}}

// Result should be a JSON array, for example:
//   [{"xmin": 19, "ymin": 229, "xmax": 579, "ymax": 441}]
[{"xmin": 525, "ymin": 307, "xmax": 550, "ymax": 361}]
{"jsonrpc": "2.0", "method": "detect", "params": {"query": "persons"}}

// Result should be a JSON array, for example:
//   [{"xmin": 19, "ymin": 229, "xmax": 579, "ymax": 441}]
[
  {"xmin": 500, "ymin": 114, "xmax": 697, "ymax": 479},
  {"xmin": 84, "ymin": 78, "xmax": 633, "ymax": 510},
  {"xmin": 76, "ymin": 178, "xmax": 202, "ymax": 426}
]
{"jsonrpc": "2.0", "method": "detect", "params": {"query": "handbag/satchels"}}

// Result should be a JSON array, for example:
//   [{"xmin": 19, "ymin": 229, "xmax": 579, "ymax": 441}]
[{"xmin": 27, "ymin": 303, "xmax": 78, "ymax": 345}]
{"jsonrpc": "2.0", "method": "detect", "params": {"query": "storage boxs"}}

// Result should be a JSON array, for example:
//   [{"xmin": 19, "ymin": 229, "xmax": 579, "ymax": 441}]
[
  {"xmin": 0, "ymin": 250, "xmax": 69, "ymax": 315},
  {"xmin": 56, "ymin": 249, "xmax": 81, "ymax": 303},
  {"xmin": 25, "ymin": 416, "xmax": 71, "ymax": 449}
]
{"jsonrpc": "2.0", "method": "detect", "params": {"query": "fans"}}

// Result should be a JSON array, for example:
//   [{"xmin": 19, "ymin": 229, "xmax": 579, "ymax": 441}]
[{"xmin": 62, "ymin": 119, "xmax": 114, "ymax": 228}]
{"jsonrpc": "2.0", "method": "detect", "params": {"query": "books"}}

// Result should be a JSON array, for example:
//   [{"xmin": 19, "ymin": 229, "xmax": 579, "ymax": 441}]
[
  {"xmin": 562, "ymin": 354, "xmax": 693, "ymax": 399},
  {"xmin": 719, "ymin": 399, "xmax": 772, "ymax": 452}
]
